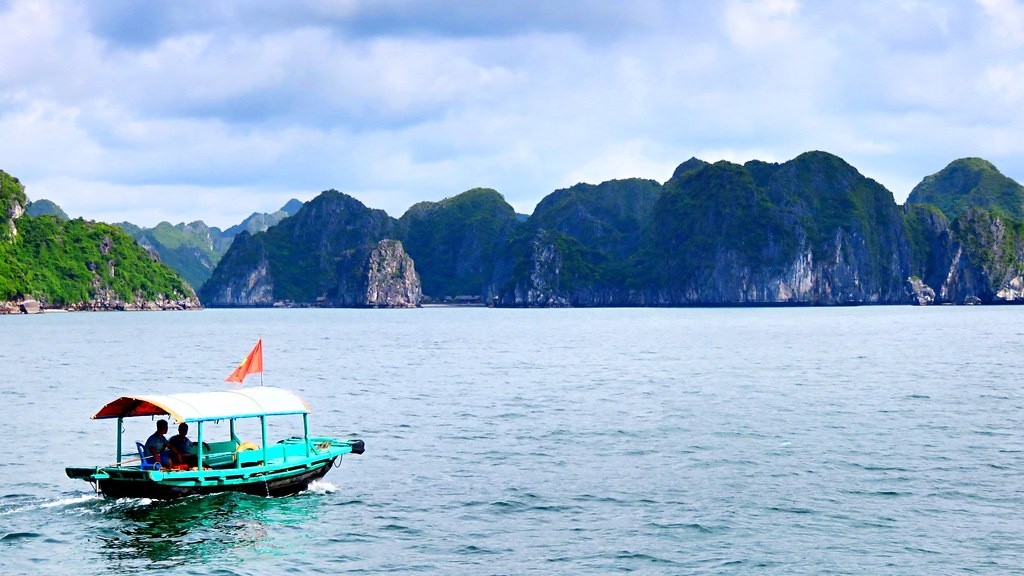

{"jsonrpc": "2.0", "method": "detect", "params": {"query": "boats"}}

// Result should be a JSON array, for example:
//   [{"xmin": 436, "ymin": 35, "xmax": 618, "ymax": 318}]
[{"xmin": 66, "ymin": 337, "xmax": 364, "ymax": 500}]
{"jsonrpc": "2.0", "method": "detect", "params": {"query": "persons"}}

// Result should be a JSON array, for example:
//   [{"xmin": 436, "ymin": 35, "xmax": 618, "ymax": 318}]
[{"xmin": 145, "ymin": 419, "xmax": 212, "ymax": 469}]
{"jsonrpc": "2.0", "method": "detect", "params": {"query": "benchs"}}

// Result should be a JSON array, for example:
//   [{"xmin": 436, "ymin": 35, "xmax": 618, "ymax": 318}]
[{"xmin": 190, "ymin": 440, "xmax": 236, "ymax": 469}]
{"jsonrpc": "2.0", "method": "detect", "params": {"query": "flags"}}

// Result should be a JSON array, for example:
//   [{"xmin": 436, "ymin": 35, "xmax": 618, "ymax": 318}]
[{"xmin": 226, "ymin": 339, "xmax": 263, "ymax": 383}]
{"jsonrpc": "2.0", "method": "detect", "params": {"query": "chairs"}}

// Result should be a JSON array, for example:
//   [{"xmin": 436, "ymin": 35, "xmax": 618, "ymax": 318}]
[{"xmin": 135, "ymin": 442, "xmax": 161, "ymax": 471}]
